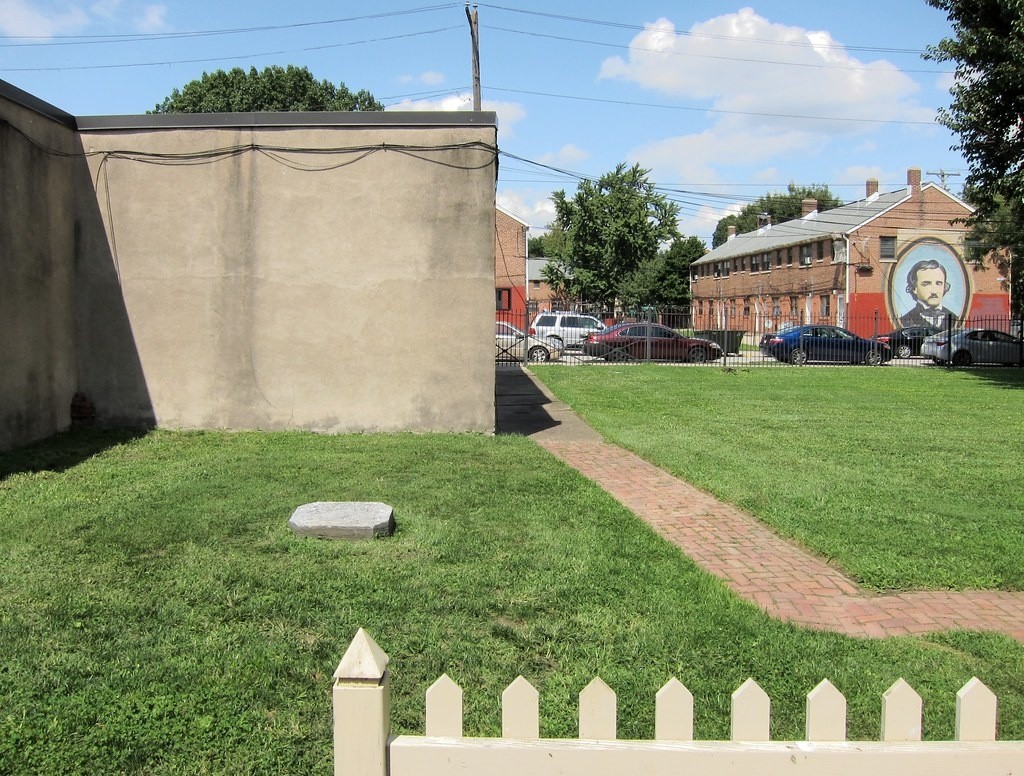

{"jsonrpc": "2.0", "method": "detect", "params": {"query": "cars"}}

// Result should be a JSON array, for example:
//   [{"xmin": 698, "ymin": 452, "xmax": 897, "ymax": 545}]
[
  {"xmin": 759, "ymin": 324, "xmax": 813, "ymax": 357},
  {"xmin": 767, "ymin": 325, "xmax": 892, "ymax": 367},
  {"xmin": 583, "ymin": 321, "xmax": 723, "ymax": 364},
  {"xmin": 872, "ymin": 327, "xmax": 946, "ymax": 359},
  {"xmin": 495, "ymin": 320, "xmax": 564, "ymax": 363},
  {"xmin": 919, "ymin": 327, "xmax": 1024, "ymax": 367}
]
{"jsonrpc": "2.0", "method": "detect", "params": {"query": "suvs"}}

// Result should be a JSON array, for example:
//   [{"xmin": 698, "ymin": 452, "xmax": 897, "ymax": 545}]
[{"xmin": 529, "ymin": 312, "xmax": 608, "ymax": 353}]
